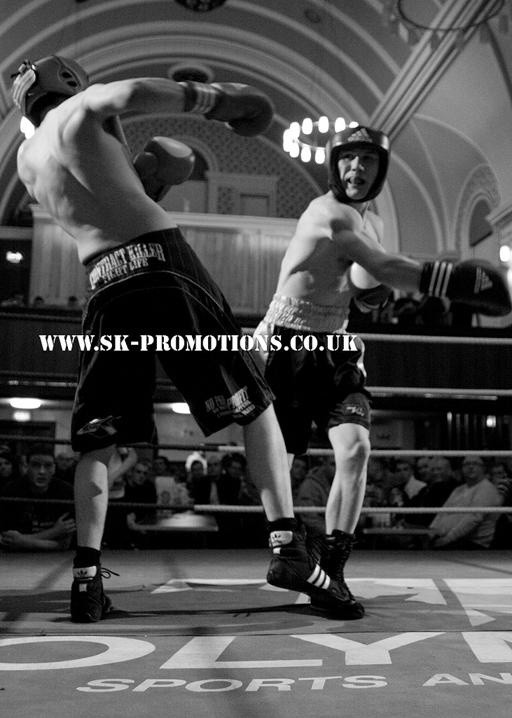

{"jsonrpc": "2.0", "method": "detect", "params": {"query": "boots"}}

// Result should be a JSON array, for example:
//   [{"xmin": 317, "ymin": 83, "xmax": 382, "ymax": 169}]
[
  {"xmin": 264, "ymin": 523, "xmax": 348, "ymax": 601},
  {"xmin": 311, "ymin": 531, "xmax": 365, "ymax": 618},
  {"xmin": 72, "ymin": 563, "xmax": 113, "ymax": 622}
]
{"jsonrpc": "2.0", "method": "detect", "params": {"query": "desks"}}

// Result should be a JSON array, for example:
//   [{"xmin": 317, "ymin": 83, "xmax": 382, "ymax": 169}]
[
  {"xmin": 356, "ymin": 513, "xmax": 433, "ymax": 550},
  {"xmin": 132, "ymin": 508, "xmax": 219, "ymax": 549}
]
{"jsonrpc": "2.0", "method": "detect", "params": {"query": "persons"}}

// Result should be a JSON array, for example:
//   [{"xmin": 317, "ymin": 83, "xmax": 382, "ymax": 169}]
[
  {"xmin": 10, "ymin": 55, "xmax": 350, "ymax": 625},
  {"xmin": 2, "ymin": 292, "xmax": 78, "ymax": 308},
  {"xmin": 249, "ymin": 122, "xmax": 512, "ymax": 622},
  {"xmin": 0, "ymin": 441, "xmax": 511, "ymax": 553}
]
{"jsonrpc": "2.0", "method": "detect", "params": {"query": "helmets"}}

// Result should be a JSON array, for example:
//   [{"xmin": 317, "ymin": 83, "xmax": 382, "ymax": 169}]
[
  {"xmin": 326, "ymin": 126, "xmax": 389, "ymax": 202},
  {"xmin": 12, "ymin": 56, "xmax": 92, "ymax": 129}
]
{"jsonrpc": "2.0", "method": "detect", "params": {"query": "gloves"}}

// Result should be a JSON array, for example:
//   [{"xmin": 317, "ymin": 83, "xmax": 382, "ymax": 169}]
[
  {"xmin": 351, "ymin": 261, "xmax": 390, "ymax": 310},
  {"xmin": 418, "ymin": 260, "xmax": 511, "ymax": 319},
  {"xmin": 180, "ymin": 79, "xmax": 272, "ymax": 138},
  {"xmin": 133, "ymin": 137, "xmax": 197, "ymax": 203}
]
